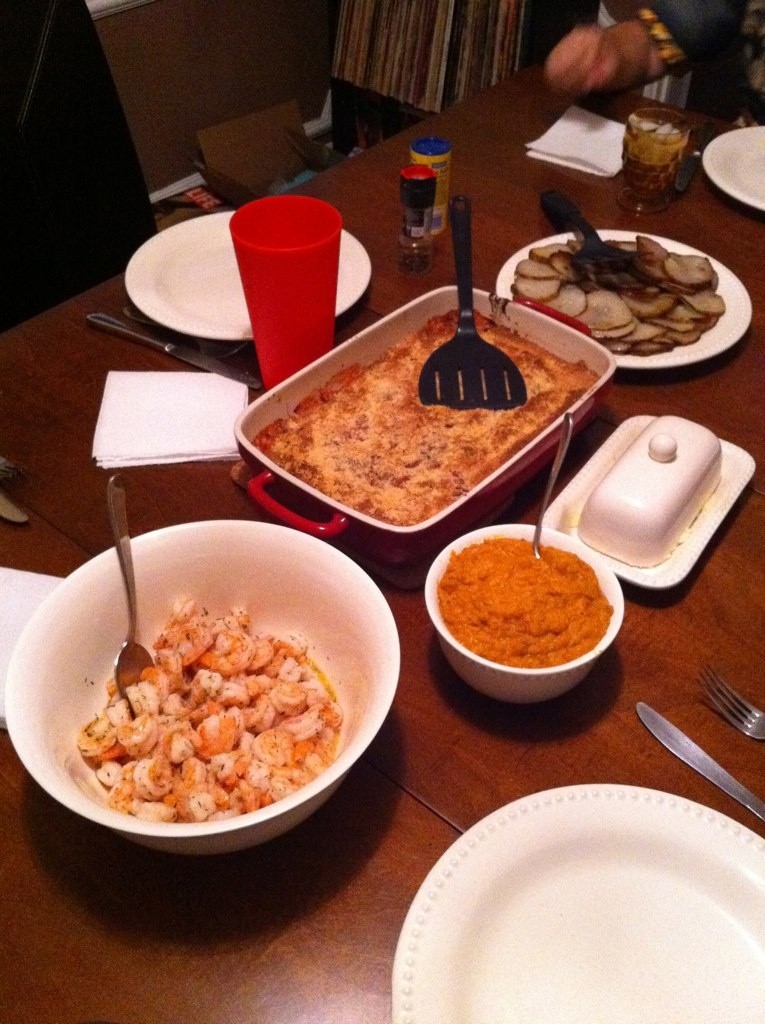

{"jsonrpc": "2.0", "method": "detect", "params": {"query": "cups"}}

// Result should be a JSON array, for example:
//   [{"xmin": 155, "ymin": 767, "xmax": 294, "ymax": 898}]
[
  {"xmin": 424, "ymin": 523, "xmax": 627, "ymax": 706},
  {"xmin": 617, "ymin": 107, "xmax": 693, "ymax": 213},
  {"xmin": 230, "ymin": 195, "xmax": 344, "ymax": 394}
]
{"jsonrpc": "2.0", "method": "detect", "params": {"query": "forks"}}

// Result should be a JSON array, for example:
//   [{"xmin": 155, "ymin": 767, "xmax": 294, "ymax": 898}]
[{"xmin": 697, "ymin": 659, "xmax": 764, "ymax": 743}]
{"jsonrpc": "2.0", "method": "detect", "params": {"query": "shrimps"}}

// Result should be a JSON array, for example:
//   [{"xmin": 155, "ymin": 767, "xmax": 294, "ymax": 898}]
[{"xmin": 77, "ymin": 594, "xmax": 344, "ymax": 825}]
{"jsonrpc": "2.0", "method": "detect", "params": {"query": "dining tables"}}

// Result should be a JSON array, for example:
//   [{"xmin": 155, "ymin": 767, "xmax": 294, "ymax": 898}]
[{"xmin": 0, "ymin": 68, "xmax": 765, "ymax": 1024}]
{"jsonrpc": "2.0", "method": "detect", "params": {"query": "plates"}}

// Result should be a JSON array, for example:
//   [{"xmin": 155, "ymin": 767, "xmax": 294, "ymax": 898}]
[
  {"xmin": 497, "ymin": 229, "xmax": 754, "ymax": 372},
  {"xmin": 545, "ymin": 414, "xmax": 757, "ymax": 588},
  {"xmin": 702, "ymin": 125, "xmax": 764, "ymax": 216},
  {"xmin": 125, "ymin": 211, "xmax": 371, "ymax": 341},
  {"xmin": 388, "ymin": 784, "xmax": 765, "ymax": 1023}
]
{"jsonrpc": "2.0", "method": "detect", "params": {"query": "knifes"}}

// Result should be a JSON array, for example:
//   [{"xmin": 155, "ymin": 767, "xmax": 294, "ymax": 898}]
[
  {"xmin": 674, "ymin": 128, "xmax": 717, "ymax": 192},
  {"xmin": 635, "ymin": 701, "xmax": 764, "ymax": 825},
  {"xmin": 89, "ymin": 310, "xmax": 263, "ymax": 391}
]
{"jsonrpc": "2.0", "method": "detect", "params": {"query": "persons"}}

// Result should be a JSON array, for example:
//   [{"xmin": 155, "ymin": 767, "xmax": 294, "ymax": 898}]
[{"xmin": 542, "ymin": 0, "xmax": 764, "ymax": 127}]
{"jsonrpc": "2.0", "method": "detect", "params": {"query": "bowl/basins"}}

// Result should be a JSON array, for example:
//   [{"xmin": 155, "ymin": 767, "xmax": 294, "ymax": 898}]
[
  {"xmin": 2, "ymin": 519, "xmax": 402, "ymax": 856},
  {"xmin": 237, "ymin": 284, "xmax": 619, "ymax": 596}
]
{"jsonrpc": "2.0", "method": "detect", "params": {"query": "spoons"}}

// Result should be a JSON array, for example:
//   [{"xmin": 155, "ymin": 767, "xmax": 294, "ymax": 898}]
[{"xmin": 106, "ymin": 473, "xmax": 158, "ymax": 713}]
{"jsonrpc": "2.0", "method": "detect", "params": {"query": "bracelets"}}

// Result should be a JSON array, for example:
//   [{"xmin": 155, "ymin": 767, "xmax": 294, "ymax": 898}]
[{"xmin": 636, "ymin": 6, "xmax": 687, "ymax": 79}]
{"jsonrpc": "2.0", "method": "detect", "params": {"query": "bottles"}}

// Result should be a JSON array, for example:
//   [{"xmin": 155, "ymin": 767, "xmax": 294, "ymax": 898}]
[{"xmin": 396, "ymin": 164, "xmax": 438, "ymax": 276}]
